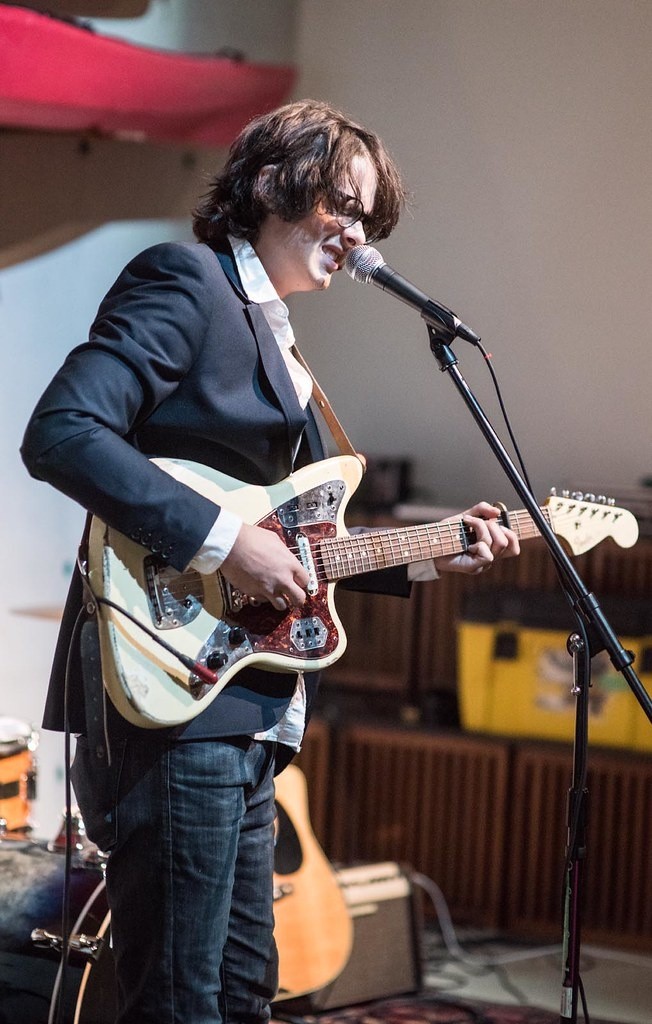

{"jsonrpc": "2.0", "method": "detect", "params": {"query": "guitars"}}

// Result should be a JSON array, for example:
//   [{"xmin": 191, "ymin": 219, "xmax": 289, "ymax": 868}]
[
  {"xmin": 87, "ymin": 453, "xmax": 640, "ymax": 731},
  {"xmin": 256, "ymin": 765, "xmax": 354, "ymax": 1003}
]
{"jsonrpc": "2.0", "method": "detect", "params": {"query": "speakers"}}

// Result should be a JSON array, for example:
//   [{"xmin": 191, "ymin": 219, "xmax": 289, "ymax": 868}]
[{"xmin": 287, "ymin": 860, "xmax": 424, "ymax": 1009}]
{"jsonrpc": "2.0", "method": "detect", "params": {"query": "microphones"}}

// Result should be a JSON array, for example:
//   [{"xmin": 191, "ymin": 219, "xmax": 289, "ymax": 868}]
[{"xmin": 345, "ymin": 245, "xmax": 481, "ymax": 346}]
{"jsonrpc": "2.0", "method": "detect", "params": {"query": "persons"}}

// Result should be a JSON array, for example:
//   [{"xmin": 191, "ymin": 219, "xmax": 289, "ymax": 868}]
[{"xmin": 19, "ymin": 97, "xmax": 523, "ymax": 1024}]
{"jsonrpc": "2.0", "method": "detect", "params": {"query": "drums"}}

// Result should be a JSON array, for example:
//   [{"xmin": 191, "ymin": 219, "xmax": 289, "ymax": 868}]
[{"xmin": 0, "ymin": 716, "xmax": 40, "ymax": 851}]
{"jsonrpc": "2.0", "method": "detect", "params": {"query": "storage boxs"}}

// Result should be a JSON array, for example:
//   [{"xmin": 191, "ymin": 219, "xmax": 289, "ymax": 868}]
[{"xmin": 454, "ymin": 598, "xmax": 652, "ymax": 751}]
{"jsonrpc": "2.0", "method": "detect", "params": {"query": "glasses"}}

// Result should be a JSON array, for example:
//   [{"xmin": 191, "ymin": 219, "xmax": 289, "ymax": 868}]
[{"xmin": 333, "ymin": 187, "xmax": 375, "ymax": 239}]
